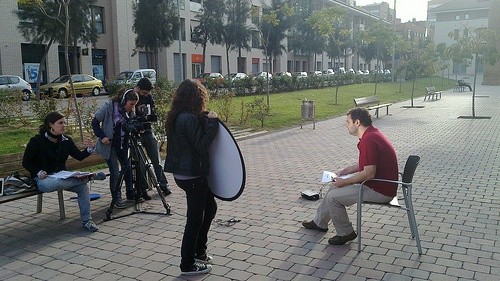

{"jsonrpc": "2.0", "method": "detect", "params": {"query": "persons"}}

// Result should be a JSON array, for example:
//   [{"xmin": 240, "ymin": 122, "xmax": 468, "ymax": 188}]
[
  {"xmin": 302, "ymin": 107, "xmax": 398, "ymax": 245},
  {"xmin": 163, "ymin": 78, "xmax": 219, "ymax": 275},
  {"xmin": 458, "ymin": 80, "xmax": 472, "ymax": 91},
  {"xmin": 129, "ymin": 77, "xmax": 172, "ymax": 200},
  {"xmin": 21, "ymin": 111, "xmax": 99, "ymax": 232},
  {"xmin": 91, "ymin": 87, "xmax": 145, "ymax": 208}
]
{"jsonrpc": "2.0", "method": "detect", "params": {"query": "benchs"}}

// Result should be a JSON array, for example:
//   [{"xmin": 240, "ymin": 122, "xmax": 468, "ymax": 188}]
[
  {"xmin": 0, "ymin": 153, "xmax": 65, "ymax": 219},
  {"xmin": 353, "ymin": 95, "xmax": 391, "ymax": 117},
  {"xmin": 456, "ymin": 82, "xmax": 465, "ymax": 92},
  {"xmin": 424, "ymin": 87, "xmax": 442, "ymax": 100}
]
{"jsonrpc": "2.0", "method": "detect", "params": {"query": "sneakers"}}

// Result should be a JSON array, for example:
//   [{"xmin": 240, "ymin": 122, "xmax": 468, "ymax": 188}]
[
  {"xmin": 195, "ymin": 253, "xmax": 213, "ymax": 263},
  {"xmin": 92, "ymin": 172, "xmax": 106, "ymax": 182},
  {"xmin": 181, "ymin": 263, "xmax": 212, "ymax": 275},
  {"xmin": 114, "ymin": 200, "xmax": 127, "ymax": 209},
  {"xmin": 127, "ymin": 195, "xmax": 145, "ymax": 203},
  {"xmin": 81, "ymin": 219, "xmax": 99, "ymax": 231}
]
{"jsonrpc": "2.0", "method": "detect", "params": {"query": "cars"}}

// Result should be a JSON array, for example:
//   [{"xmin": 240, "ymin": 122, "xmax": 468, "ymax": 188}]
[
  {"xmin": 294, "ymin": 71, "xmax": 308, "ymax": 81},
  {"xmin": 323, "ymin": 67, "xmax": 392, "ymax": 75},
  {"xmin": 223, "ymin": 72, "xmax": 249, "ymax": 87},
  {"xmin": 195, "ymin": 72, "xmax": 223, "ymax": 89},
  {"xmin": 250, "ymin": 72, "xmax": 272, "ymax": 85},
  {"xmin": 275, "ymin": 72, "xmax": 292, "ymax": 81},
  {"xmin": 34, "ymin": 74, "xmax": 103, "ymax": 99},
  {"xmin": 308, "ymin": 71, "xmax": 322, "ymax": 76},
  {"xmin": 0, "ymin": 75, "xmax": 33, "ymax": 102}
]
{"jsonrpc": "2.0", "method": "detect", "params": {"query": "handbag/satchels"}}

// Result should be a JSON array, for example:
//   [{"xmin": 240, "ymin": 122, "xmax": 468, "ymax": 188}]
[
  {"xmin": 3, "ymin": 172, "xmax": 37, "ymax": 195},
  {"xmin": 301, "ymin": 190, "xmax": 319, "ymax": 199}
]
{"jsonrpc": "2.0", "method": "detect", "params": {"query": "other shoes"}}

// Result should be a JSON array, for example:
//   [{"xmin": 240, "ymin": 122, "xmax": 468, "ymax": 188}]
[
  {"xmin": 302, "ymin": 219, "xmax": 328, "ymax": 232},
  {"xmin": 144, "ymin": 192, "xmax": 151, "ymax": 200},
  {"xmin": 328, "ymin": 231, "xmax": 357, "ymax": 245},
  {"xmin": 163, "ymin": 187, "xmax": 172, "ymax": 195}
]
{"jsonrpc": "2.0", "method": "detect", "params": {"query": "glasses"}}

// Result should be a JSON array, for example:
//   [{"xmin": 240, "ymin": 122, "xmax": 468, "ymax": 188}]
[{"xmin": 141, "ymin": 90, "xmax": 150, "ymax": 94}]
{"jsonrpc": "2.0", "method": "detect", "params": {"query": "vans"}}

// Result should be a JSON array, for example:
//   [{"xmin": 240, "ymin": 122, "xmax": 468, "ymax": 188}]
[{"xmin": 104, "ymin": 69, "xmax": 158, "ymax": 92}]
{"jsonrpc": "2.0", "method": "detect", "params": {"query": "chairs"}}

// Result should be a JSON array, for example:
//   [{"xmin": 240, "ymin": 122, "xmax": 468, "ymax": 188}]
[{"xmin": 357, "ymin": 155, "xmax": 421, "ymax": 252}]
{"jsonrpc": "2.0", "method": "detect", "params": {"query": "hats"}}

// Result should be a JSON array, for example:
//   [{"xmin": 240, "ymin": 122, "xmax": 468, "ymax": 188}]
[{"xmin": 139, "ymin": 78, "xmax": 153, "ymax": 89}]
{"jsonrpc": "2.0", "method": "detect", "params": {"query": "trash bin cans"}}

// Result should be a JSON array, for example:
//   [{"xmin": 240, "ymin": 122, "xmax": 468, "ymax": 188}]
[{"xmin": 300, "ymin": 100, "xmax": 315, "ymax": 130}]
